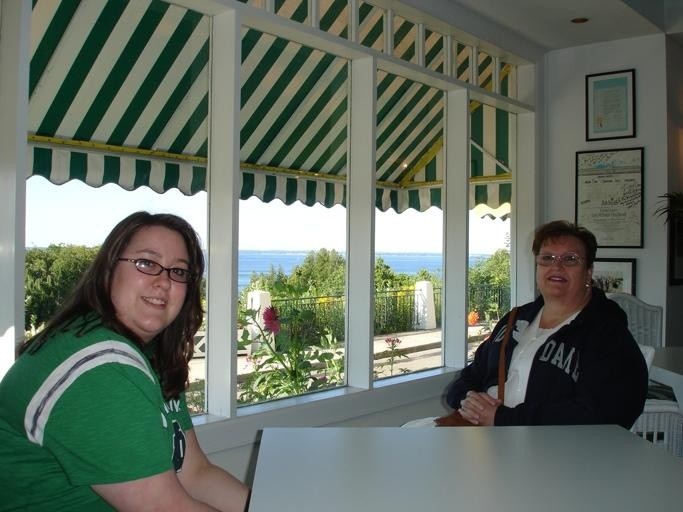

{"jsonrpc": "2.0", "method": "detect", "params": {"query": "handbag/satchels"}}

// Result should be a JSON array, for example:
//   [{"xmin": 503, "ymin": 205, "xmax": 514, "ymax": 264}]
[{"xmin": 433, "ymin": 306, "xmax": 516, "ymax": 427}]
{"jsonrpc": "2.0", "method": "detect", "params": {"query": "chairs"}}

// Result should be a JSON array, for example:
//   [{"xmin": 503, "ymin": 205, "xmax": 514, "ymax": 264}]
[
  {"xmin": 602, "ymin": 292, "xmax": 662, "ymax": 350},
  {"xmin": 629, "ymin": 402, "xmax": 683, "ymax": 457}
]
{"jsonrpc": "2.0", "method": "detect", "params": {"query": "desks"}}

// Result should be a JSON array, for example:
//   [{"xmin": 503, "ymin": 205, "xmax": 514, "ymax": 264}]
[{"xmin": 245, "ymin": 424, "xmax": 683, "ymax": 512}]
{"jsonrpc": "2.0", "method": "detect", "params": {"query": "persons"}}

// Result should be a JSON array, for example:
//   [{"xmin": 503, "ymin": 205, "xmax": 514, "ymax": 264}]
[
  {"xmin": 400, "ymin": 220, "xmax": 650, "ymax": 430},
  {"xmin": 0, "ymin": 211, "xmax": 250, "ymax": 512}
]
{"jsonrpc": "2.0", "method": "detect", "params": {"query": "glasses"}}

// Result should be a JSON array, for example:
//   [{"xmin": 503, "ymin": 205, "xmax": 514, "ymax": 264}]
[
  {"xmin": 117, "ymin": 256, "xmax": 194, "ymax": 284},
  {"xmin": 535, "ymin": 252, "xmax": 581, "ymax": 266}
]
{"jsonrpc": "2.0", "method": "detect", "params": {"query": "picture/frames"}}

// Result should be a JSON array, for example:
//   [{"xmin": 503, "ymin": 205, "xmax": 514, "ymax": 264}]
[
  {"xmin": 585, "ymin": 69, "xmax": 636, "ymax": 141},
  {"xmin": 575, "ymin": 147, "xmax": 645, "ymax": 249},
  {"xmin": 590, "ymin": 258, "xmax": 636, "ymax": 297}
]
